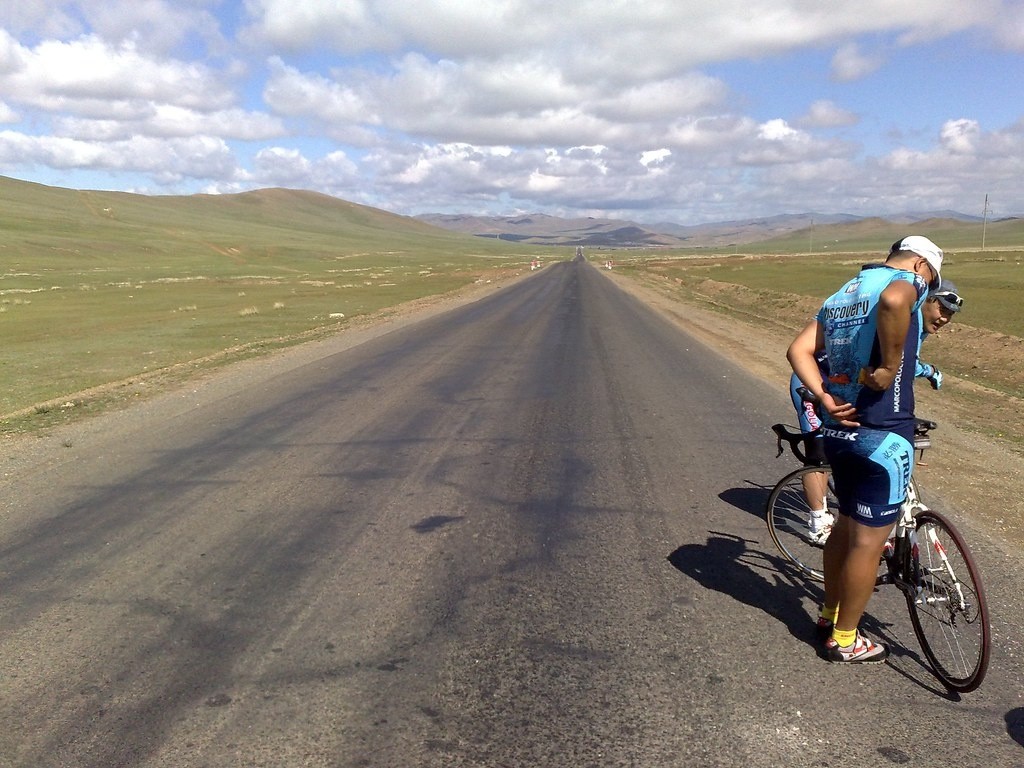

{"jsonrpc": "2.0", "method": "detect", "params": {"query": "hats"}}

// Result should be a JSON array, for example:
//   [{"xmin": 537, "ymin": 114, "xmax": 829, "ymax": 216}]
[
  {"xmin": 889, "ymin": 235, "xmax": 943, "ymax": 297},
  {"xmin": 928, "ymin": 280, "xmax": 961, "ymax": 312}
]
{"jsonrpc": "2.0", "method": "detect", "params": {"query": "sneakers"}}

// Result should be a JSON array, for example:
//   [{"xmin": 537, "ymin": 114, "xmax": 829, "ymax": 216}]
[
  {"xmin": 817, "ymin": 617, "xmax": 886, "ymax": 665},
  {"xmin": 808, "ymin": 525, "xmax": 833, "ymax": 545}
]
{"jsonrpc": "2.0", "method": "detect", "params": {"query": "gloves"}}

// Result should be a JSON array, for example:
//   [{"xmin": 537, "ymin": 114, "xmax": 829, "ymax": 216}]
[{"xmin": 927, "ymin": 365, "xmax": 942, "ymax": 390}]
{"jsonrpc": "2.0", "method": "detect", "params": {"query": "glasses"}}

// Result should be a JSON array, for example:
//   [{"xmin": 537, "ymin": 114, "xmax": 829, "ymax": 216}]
[
  {"xmin": 926, "ymin": 263, "xmax": 937, "ymax": 290},
  {"xmin": 935, "ymin": 292, "xmax": 964, "ymax": 306}
]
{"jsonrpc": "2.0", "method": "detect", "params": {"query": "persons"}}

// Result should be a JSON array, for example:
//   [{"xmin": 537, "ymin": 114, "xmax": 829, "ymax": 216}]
[
  {"xmin": 785, "ymin": 234, "xmax": 944, "ymax": 664},
  {"xmin": 788, "ymin": 278, "xmax": 965, "ymax": 549}
]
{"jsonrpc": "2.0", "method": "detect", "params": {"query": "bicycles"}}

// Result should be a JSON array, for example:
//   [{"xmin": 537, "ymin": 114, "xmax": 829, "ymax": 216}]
[{"xmin": 766, "ymin": 419, "xmax": 991, "ymax": 696}]
